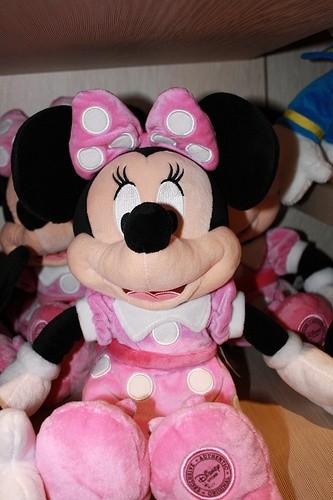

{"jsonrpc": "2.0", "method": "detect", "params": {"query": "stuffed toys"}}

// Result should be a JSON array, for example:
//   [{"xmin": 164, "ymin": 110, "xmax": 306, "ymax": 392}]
[{"xmin": 0, "ymin": 43, "xmax": 333, "ymax": 500}]
